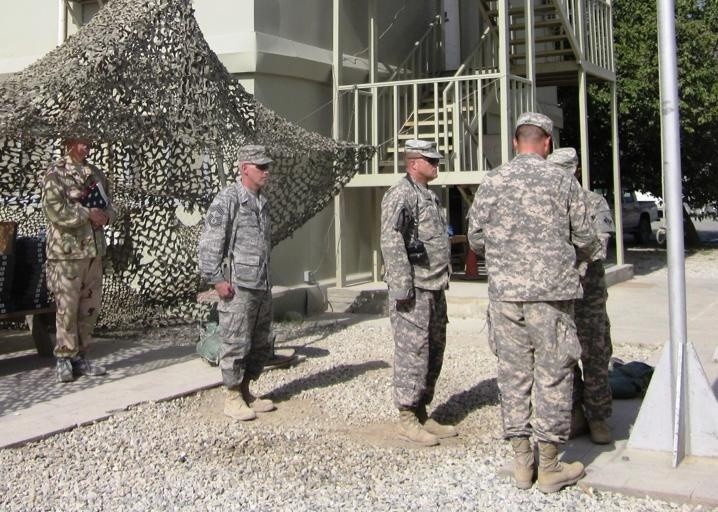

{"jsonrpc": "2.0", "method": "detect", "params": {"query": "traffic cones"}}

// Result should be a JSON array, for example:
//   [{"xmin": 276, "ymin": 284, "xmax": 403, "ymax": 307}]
[{"xmin": 457, "ymin": 245, "xmax": 482, "ymax": 281}]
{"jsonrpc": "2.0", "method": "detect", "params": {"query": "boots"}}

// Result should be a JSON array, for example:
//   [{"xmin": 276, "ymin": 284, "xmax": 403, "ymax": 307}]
[
  {"xmin": 397, "ymin": 409, "xmax": 441, "ymax": 446},
  {"xmin": 244, "ymin": 383, "xmax": 275, "ymax": 413},
  {"xmin": 418, "ymin": 405, "xmax": 458, "ymax": 438},
  {"xmin": 224, "ymin": 388, "xmax": 255, "ymax": 421},
  {"xmin": 509, "ymin": 406, "xmax": 612, "ymax": 494}
]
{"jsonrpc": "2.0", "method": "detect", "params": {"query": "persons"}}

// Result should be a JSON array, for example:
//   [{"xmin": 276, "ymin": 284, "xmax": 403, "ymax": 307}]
[
  {"xmin": 465, "ymin": 111, "xmax": 603, "ymax": 494},
  {"xmin": 546, "ymin": 146, "xmax": 616, "ymax": 445},
  {"xmin": 42, "ymin": 124, "xmax": 119, "ymax": 383},
  {"xmin": 379, "ymin": 139, "xmax": 458, "ymax": 447},
  {"xmin": 198, "ymin": 144, "xmax": 275, "ymax": 421}
]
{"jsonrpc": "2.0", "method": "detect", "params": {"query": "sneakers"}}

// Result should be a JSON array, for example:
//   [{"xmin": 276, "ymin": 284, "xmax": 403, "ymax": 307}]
[
  {"xmin": 75, "ymin": 358, "xmax": 106, "ymax": 375},
  {"xmin": 57, "ymin": 356, "xmax": 73, "ymax": 381}
]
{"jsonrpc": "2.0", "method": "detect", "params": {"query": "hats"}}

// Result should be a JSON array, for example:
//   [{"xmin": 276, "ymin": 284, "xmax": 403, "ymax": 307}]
[
  {"xmin": 404, "ymin": 140, "xmax": 444, "ymax": 160},
  {"xmin": 547, "ymin": 147, "xmax": 580, "ymax": 175},
  {"xmin": 60, "ymin": 120, "xmax": 96, "ymax": 142},
  {"xmin": 514, "ymin": 112, "xmax": 555, "ymax": 153},
  {"xmin": 237, "ymin": 146, "xmax": 273, "ymax": 166}
]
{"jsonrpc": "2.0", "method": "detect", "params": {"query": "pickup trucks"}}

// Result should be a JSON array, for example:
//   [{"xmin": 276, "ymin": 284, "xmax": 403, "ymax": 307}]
[{"xmin": 591, "ymin": 189, "xmax": 659, "ymax": 245}]
